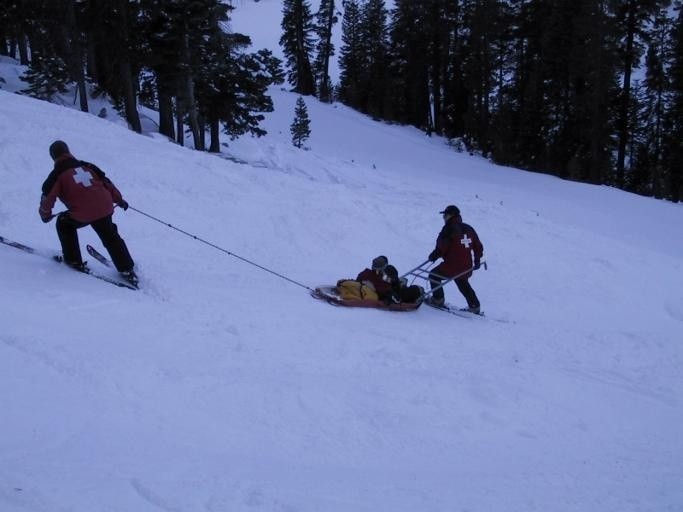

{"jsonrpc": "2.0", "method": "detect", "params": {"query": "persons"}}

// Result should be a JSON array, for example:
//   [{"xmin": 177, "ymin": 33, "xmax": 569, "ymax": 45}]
[
  {"xmin": 39, "ymin": 140, "xmax": 138, "ymax": 285},
  {"xmin": 427, "ymin": 205, "xmax": 483, "ymax": 313}
]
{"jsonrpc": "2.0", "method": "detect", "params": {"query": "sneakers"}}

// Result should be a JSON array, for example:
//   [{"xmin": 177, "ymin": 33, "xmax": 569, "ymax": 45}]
[
  {"xmin": 427, "ymin": 296, "xmax": 444, "ymax": 305},
  {"xmin": 121, "ymin": 270, "xmax": 138, "ymax": 282},
  {"xmin": 465, "ymin": 306, "xmax": 480, "ymax": 314}
]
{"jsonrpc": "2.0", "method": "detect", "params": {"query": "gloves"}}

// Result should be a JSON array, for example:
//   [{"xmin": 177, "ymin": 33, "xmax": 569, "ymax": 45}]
[
  {"xmin": 118, "ymin": 199, "xmax": 128, "ymax": 211},
  {"xmin": 428, "ymin": 252, "xmax": 440, "ymax": 263},
  {"xmin": 473, "ymin": 261, "xmax": 480, "ymax": 270},
  {"xmin": 42, "ymin": 216, "xmax": 51, "ymax": 223}
]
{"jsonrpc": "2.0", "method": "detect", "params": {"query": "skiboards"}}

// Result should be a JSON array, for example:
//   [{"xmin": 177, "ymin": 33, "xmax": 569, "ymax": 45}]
[{"xmin": 0, "ymin": 236, "xmax": 140, "ymax": 291}]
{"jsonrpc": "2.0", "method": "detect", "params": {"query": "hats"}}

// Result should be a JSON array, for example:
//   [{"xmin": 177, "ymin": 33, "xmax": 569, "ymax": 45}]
[{"xmin": 440, "ymin": 205, "xmax": 460, "ymax": 215}]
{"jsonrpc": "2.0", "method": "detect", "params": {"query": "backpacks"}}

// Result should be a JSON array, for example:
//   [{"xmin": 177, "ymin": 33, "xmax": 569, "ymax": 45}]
[{"xmin": 336, "ymin": 256, "xmax": 425, "ymax": 304}]
